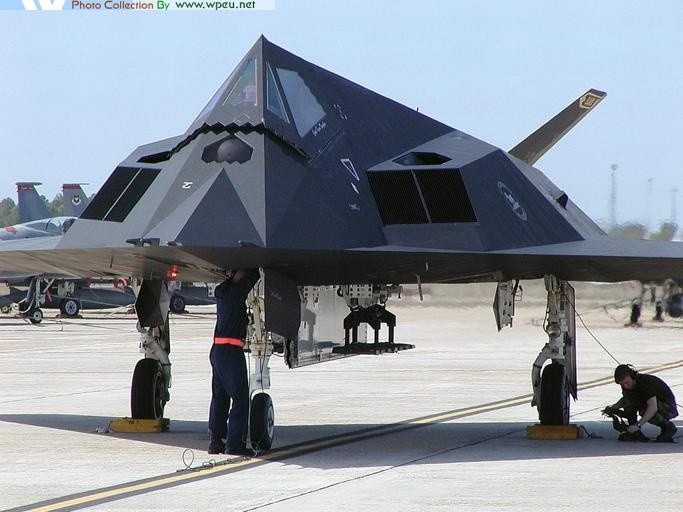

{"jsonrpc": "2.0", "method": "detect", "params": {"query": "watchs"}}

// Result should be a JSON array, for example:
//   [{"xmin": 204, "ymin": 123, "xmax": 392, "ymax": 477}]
[{"xmin": 636, "ymin": 422, "xmax": 642, "ymax": 429}]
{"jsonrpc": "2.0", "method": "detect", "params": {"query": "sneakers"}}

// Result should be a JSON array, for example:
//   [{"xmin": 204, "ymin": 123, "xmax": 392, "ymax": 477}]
[
  {"xmin": 224, "ymin": 447, "xmax": 252, "ymax": 456},
  {"xmin": 655, "ymin": 420, "xmax": 679, "ymax": 442},
  {"xmin": 617, "ymin": 431, "xmax": 643, "ymax": 442},
  {"xmin": 207, "ymin": 439, "xmax": 225, "ymax": 454}
]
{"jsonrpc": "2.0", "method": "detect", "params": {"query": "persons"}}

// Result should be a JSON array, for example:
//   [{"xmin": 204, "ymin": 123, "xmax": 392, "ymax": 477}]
[
  {"xmin": 208, "ymin": 268, "xmax": 260, "ymax": 456},
  {"xmin": 600, "ymin": 364, "xmax": 678, "ymax": 443}
]
